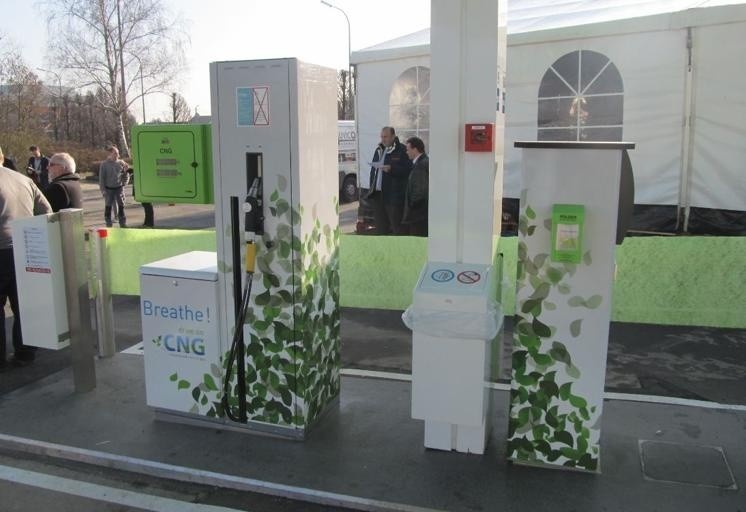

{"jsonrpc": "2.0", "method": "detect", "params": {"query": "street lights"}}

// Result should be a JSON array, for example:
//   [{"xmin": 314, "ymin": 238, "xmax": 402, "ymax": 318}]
[
  {"xmin": 319, "ymin": 0, "xmax": 353, "ymax": 118},
  {"xmin": 35, "ymin": 66, "xmax": 61, "ymax": 102},
  {"xmin": 113, "ymin": 47, "xmax": 145, "ymax": 124}
]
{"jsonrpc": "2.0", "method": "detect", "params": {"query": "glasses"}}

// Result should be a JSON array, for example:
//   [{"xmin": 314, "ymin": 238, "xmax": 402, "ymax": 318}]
[{"xmin": 49, "ymin": 162, "xmax": 65, "ymax": 167}]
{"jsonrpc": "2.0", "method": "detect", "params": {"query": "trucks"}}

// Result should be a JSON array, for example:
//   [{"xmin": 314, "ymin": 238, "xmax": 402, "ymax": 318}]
[{"xmin": 338, "ymin": 120, "xmax": 361, "ymax": 202}]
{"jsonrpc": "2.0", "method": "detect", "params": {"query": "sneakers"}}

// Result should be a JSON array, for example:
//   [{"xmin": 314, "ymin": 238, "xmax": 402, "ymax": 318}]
[
  {"xmin": 0, "ymin": 352, "xmax": 33, "ymax": 373},
  {"xmin": 139, "ymin": 225, "xmax": 153, "ymax": 229}
]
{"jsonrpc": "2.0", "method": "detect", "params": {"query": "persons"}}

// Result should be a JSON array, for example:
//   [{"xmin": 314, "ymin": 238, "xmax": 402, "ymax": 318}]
[
  {"xmin": 3, "ymin": 153, "xmax": 16, "ymax": 170},
  {"xmin": 378, "ymin": 137, "xmax": 429, "ymax": 236},
  {"xmin": 98, "ymin": 146, "xmax": 129, "ymax": 228},
  {"xmin": 0, "ymin": 150, "xmax": 54, "ymax": 369},
  {"xmin": 127, "ymin": 168, "xmax": 155, "ymax": 228},
  {"xmin": 26, "ymin": 145, "xmax": 50, "ymax": 196},
  {"xmin": 107, "ymin": 155, "xmax": 125, "ymax": 223},
  {"xmin": 369, "ymin": 126, "xmax": 411, "ymax": 234},
  {"xmin": 45, "ymin": 152, "xmax": 82, "ymax": 212}
]
